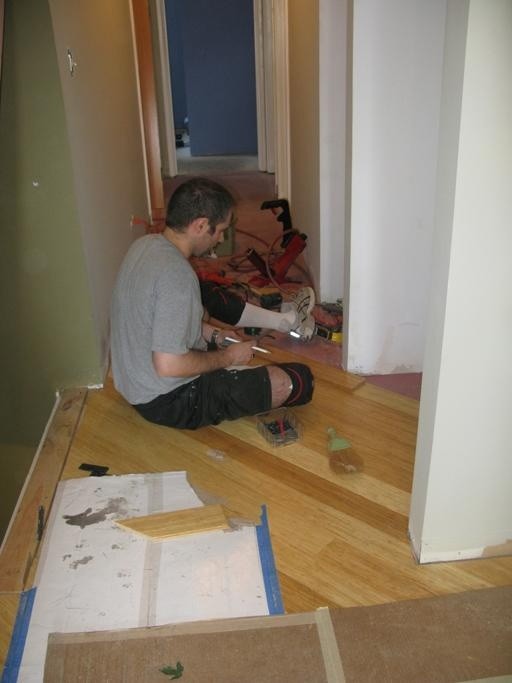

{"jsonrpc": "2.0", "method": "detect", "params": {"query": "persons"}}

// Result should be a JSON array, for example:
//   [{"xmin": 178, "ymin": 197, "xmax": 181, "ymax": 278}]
[
  {"xmin": 147, "ymin": 173, "xmax": 315, "ymax": 335},
  {"xmin": 108, "ymin": 178, "xmax": 314, "ymax": 430}
]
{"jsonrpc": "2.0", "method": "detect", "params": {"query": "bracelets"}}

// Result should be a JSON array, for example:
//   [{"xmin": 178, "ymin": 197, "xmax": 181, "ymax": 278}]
[{"xmin": 211, "ymin": 329, "xmax": 222, "ymax": 345}]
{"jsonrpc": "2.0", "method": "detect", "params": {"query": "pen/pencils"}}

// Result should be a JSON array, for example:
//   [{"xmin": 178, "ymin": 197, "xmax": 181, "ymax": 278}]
[{"xmin": 225, "ymin": 335, "xmax": 272, "ymax": 356}]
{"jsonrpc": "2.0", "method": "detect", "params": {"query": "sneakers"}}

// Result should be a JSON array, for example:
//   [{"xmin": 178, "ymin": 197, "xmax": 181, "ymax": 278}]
[{"xmin": 288, "ymin": 286, "xmax": 316, "ymax": 344}]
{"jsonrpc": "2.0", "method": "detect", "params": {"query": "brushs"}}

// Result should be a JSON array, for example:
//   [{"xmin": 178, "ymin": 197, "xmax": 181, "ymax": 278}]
[{"xmin": 326, "ymin": 427, "xmax": 363, "ymax": 473}]
